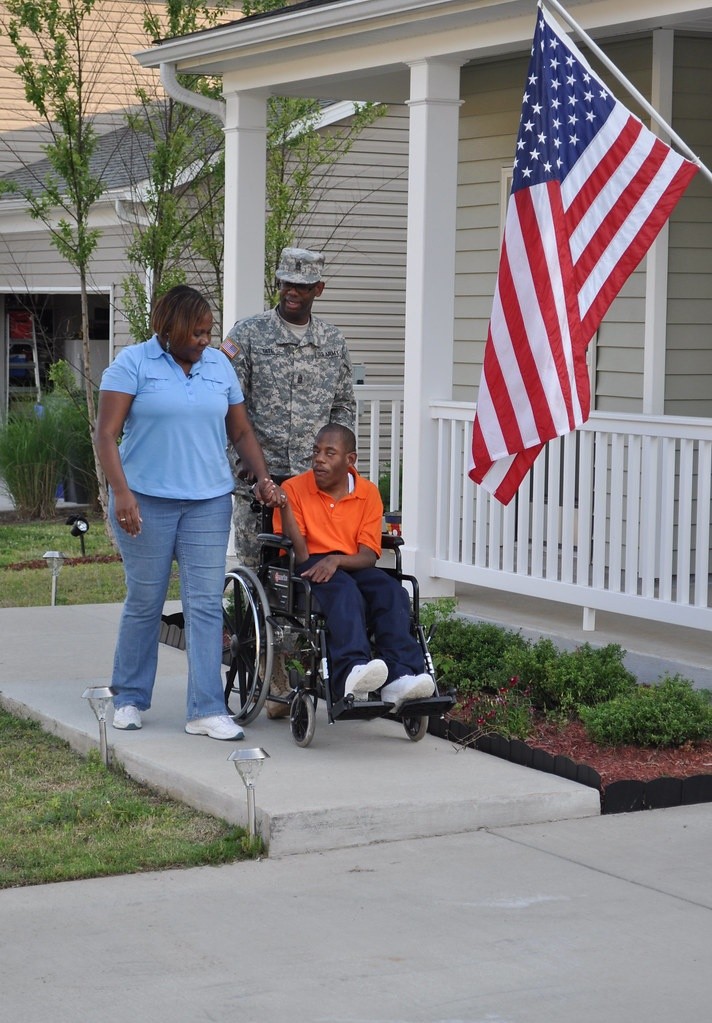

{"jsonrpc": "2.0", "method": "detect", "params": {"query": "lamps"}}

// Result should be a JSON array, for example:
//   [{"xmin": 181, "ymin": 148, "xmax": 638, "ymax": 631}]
[
  {"xmin": 41, "ymin": 551, "xmax": 69, "ymax": 574},
  {"xmin": 67, "ymin": 515, "xmax": 89, "ymax": 536},
  {"xmin": 228, "ymin": 747, "xmax": 271, "ymax": 787},
  {"xmin": 80, "ymin": 686, "xmax": 120, "ymax": 721}
]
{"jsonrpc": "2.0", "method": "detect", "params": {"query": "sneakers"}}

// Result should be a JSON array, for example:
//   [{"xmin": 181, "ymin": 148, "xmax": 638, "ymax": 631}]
[
  {"xmin": 112, "ymin": 705, "xmax": 143, "ymax": 729},
  {"xmin": 184, "ymin": 715, "xmax": 245, "ymax": 740},
  {"xmin": 382, "ymin": 673, "xmax": 434, "ymax": 714},
  {"xmin": 343, "ymin": 659, "xmax": 388, "ymax": 702}
]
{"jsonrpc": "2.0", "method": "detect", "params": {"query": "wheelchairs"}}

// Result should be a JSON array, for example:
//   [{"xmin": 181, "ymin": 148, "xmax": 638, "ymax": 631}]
[{"xmin": 215, "ymin": 494, "xmax": 458, "ymax": 748}]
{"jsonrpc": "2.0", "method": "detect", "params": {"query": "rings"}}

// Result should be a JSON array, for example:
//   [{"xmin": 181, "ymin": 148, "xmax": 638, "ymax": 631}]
[
  {"xmin": 281, "ymin": 495, "xmax": 285, "ymax": 498},
  {"xmin": 120, "ymin": 518, "xmax": 125, "ymax": 521}
]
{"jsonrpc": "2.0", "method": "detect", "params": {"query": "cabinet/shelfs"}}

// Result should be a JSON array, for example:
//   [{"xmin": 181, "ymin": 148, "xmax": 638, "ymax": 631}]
[{"xmin": 5, "ymin": 294, "xmax": 55, "ymax": 369}]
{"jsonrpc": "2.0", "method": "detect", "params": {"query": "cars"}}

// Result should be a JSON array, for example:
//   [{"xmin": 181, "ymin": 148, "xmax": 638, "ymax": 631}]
[{"xmin": 8, "ymin": 344, "xmax": 34, "ymax": 387}]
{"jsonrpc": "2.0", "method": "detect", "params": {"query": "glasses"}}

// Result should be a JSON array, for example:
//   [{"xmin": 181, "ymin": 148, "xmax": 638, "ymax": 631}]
[{"xmin": 276, "ymin": 279, "xmax": 319, "ymax": 294}]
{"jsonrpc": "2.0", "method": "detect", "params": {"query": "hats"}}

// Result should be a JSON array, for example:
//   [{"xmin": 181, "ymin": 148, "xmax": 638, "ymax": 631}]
[{"xmin": 274, "ymin": 248, "xmax": 325, "ymax": 284}]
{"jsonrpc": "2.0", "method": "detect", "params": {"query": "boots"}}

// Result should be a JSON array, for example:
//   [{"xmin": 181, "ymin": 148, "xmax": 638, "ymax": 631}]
[{"xmin": 256, "ymin": 653, "xmax": 300, "ymax": 719}]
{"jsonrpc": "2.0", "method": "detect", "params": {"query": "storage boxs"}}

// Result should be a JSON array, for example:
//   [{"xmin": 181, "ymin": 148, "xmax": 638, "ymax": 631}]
[
  {"xmin": 9, "ymin": 311, "xmax": 33, "ymax": 338},
  {"xmin": 10, "ymin": 353, "xmax": 26, "ymax": 377}
]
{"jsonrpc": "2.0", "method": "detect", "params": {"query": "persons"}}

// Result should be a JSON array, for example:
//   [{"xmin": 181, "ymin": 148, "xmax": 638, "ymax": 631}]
[
  {"xmin": 93, "ymin": 284, "xmax": 288, "ymax": 741},
  {"xmin": 261, "ymin": 423, "xmax": 436, "ymax": 713},
  {"xmin": 220, "ymin": 248, "xmax": 357, "ymax": 718}
]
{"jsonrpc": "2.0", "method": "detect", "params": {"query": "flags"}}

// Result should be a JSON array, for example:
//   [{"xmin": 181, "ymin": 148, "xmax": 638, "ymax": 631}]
[{"xmin": 467, "ymin": 0, "xmax": 698, "ymax": 504}]
